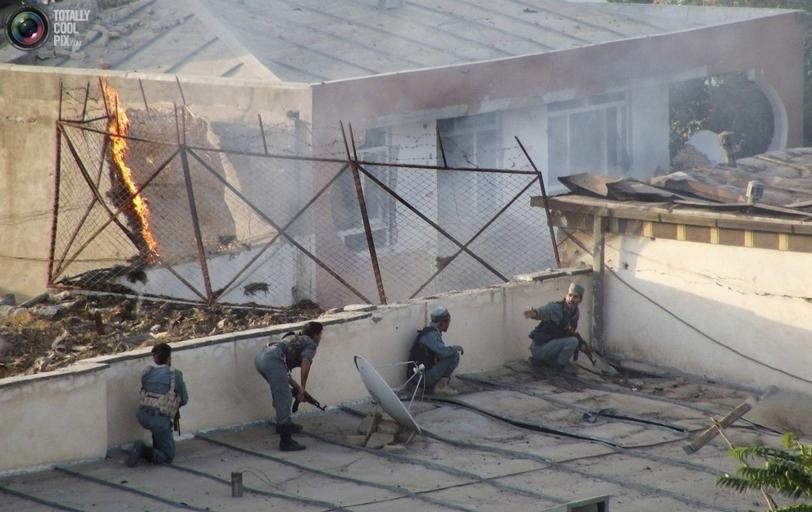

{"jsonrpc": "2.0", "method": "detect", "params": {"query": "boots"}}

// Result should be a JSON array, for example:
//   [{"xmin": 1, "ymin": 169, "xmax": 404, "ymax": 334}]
[
  {"xmin": 550, "ymin": 361, "xmax": 573, "ymax": 389},
  {"xmin": 126, "ymin": 439, "xmax": 154, "ymax": 468},
  {"xmin": 276, "ymin": 417, "xmax": 302, "ymax": 433},
  {"xmin": 434, "ymin": 375, "xmax": 459, "ymax": 396},
  {"xmin": 279, "ymin": 425, "xmax": 307, "ymax": 451}
]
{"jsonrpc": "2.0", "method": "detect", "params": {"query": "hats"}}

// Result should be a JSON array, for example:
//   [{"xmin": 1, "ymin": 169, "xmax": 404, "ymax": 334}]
[
  {"xmin": 428, "ymin": 307, "xmax": 449, "ymax": 327},
  {"xmin": 569, "ymin": 282, "xmax": 586, "ymax": 297}
]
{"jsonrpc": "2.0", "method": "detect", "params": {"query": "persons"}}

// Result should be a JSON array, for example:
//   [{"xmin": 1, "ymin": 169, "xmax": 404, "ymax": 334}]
[
  {"xmin": 407, "ymin": 307, "xmax": 463, "ymax": 397},
  {"xmin": 525, "ymin": 283, "xmax": 584, "ymax": 376},
  {"xmin": 128, "ymin": 344, "xmax": 189, "ymax": 468},
  {"xmin": 255, "ymin": 322, "xmax": 323, "ymax": 451}
]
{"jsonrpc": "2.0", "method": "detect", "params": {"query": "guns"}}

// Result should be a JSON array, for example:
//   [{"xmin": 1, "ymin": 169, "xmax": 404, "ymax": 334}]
[
  {"xmin": 289, "ymin": 377, "xmax": 327, "ymax": 413},
  {"xmin": 571, "ymin": 333, "xmax": 597, "ymax": 366},
  {"xmin": 173, "ymin": 410, "xmax": 181, "ymax": 436}
]
